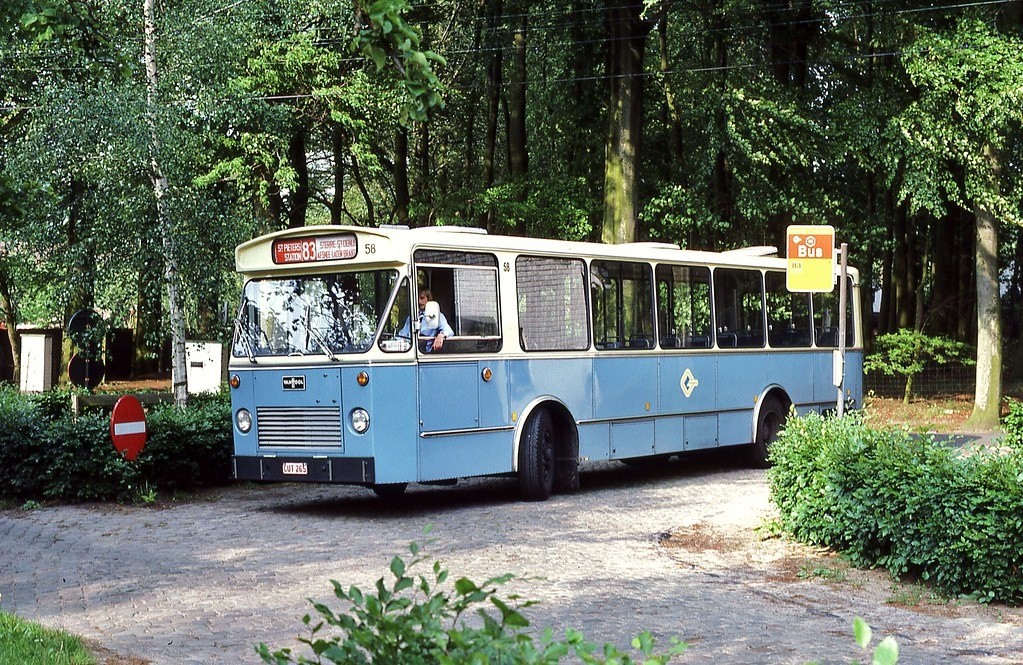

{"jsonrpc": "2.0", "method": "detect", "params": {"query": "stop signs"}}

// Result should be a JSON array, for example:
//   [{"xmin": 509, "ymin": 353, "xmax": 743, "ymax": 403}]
[{"xmin": 110, "ymin": 395, "xmax": 147, "ymax": 462}]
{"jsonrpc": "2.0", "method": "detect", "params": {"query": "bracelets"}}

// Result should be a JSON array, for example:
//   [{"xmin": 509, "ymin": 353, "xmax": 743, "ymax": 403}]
[{"xmin": 442, "ymin": 333, "xmax": 446, "ymax": 338}]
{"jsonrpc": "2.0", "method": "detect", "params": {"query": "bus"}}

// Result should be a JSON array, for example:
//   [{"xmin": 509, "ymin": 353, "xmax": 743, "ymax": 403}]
[{"xmin": 227, "ymin": 225, "xmax": 864, "ymax": 501}]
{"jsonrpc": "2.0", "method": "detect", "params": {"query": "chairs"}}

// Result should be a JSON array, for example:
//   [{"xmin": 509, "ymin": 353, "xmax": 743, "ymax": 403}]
[{"xmin": 594, "ymin": 324, "xmax": 853, "ymax": 349}]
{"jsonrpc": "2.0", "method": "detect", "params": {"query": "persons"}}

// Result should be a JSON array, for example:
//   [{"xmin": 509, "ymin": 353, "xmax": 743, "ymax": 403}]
[{"xmin": 399, "ymin": 288, "xmax": 454, "ymax": 352}]
